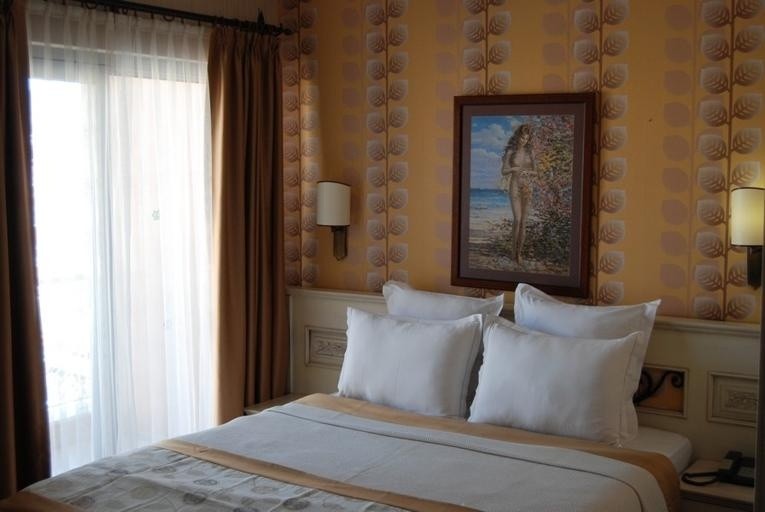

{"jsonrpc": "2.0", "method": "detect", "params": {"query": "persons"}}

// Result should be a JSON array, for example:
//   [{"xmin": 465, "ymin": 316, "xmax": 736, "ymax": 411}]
[{"xmin": 499, "ymin": 125, "xmax": 539, "ymax": 267}]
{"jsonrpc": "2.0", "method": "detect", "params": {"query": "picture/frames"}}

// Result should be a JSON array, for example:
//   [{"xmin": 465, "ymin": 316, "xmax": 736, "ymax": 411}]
[{"xmin": 451, "ymin": 92, "xmax": 599, "ymax": 299}]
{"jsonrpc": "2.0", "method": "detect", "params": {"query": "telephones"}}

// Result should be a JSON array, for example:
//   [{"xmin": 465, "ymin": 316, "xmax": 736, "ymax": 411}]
[{"xmin": 715, "ymin": 451, "xmax": 754, "ymax": 487}]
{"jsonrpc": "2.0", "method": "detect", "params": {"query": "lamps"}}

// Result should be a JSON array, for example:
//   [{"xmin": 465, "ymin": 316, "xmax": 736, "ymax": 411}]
[
  {"xmin": 316, "ymin": 180, "xmax": 351, "ymax": 262},
  {"xmin": 729, "ymin": 187, "xmax": 765, "ymax": 291}
]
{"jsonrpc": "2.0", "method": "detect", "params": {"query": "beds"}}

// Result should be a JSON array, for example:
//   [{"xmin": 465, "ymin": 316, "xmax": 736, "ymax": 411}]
[{"xmin": 0, "ymin": 392, "xmax": 691, "ymax": 511}]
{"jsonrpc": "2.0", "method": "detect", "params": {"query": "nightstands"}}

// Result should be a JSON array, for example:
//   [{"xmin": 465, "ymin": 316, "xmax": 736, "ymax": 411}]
[{"xmin": 677, "ymin": 455, "xmax": 756, "ymax": 510}]
